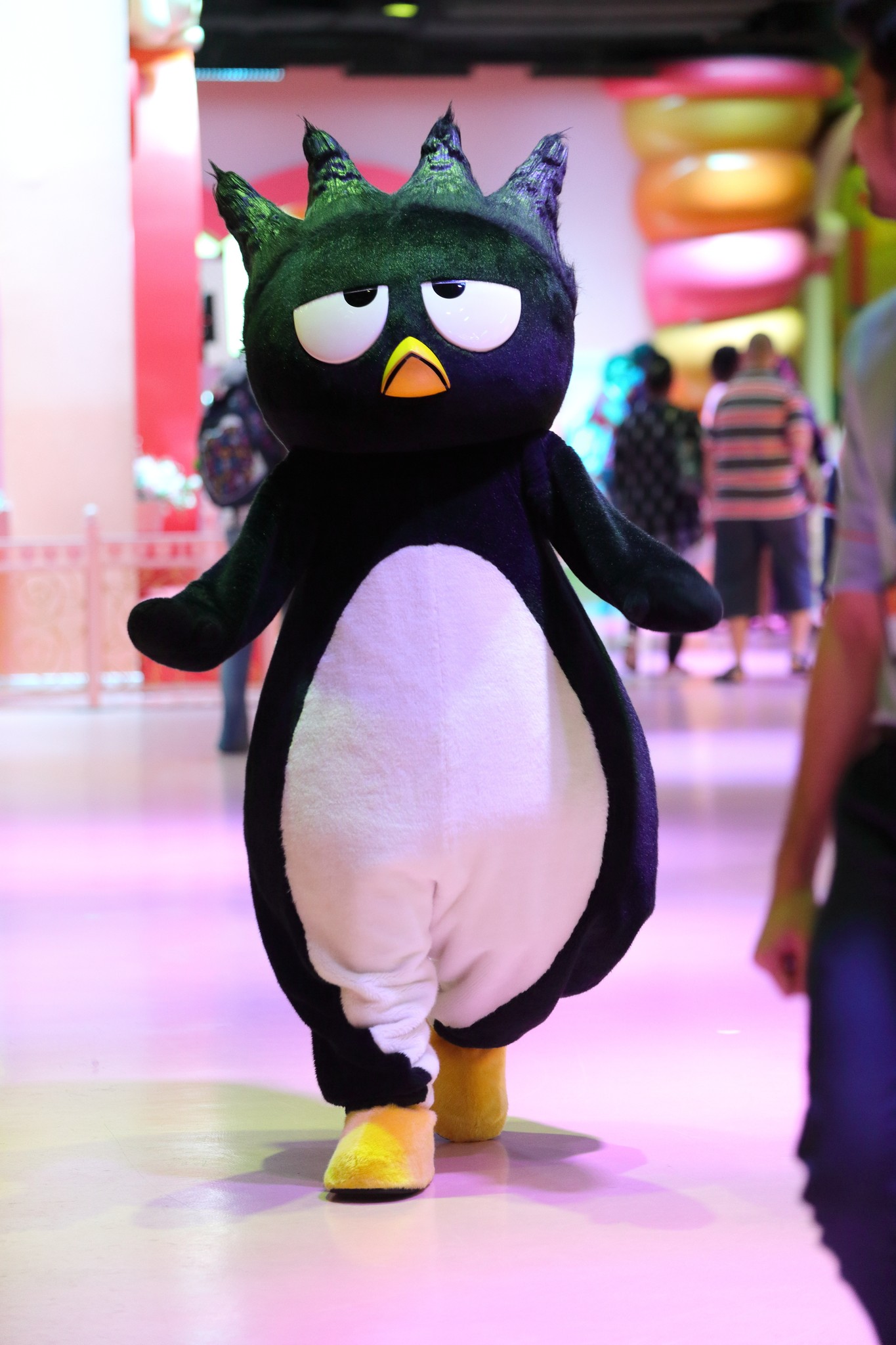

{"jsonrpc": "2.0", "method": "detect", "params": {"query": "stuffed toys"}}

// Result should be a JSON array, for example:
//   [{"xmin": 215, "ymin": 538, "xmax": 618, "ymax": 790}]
[{"xmin": 125, "ymin": 103, "xmax": 719, "ymax": 1196}]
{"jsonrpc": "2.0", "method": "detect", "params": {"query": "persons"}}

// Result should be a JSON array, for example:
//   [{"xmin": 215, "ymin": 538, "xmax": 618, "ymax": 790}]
[
  {"xmin": 757, "ymin": 0, "xmax": 896, "ymax": 1343},
  {"xmin": 207, "ymin": 331, "xmax": 839, "ymax": 752}
]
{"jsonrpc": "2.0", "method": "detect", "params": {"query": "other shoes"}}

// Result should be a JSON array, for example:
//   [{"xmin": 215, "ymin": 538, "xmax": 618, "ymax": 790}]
[
  {"xmin": 792, "ymin": 663, "xmax": 815, "ymax": 674},
  {"xmin": 715, "ymin": 671, "xmax": 746, "ymax": 682}
]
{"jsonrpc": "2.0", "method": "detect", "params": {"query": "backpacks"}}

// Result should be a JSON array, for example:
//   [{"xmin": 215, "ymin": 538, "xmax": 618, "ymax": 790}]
[{"xmin": 198, "ymin": 377, "xmax": 290, "ymax": 506}]
